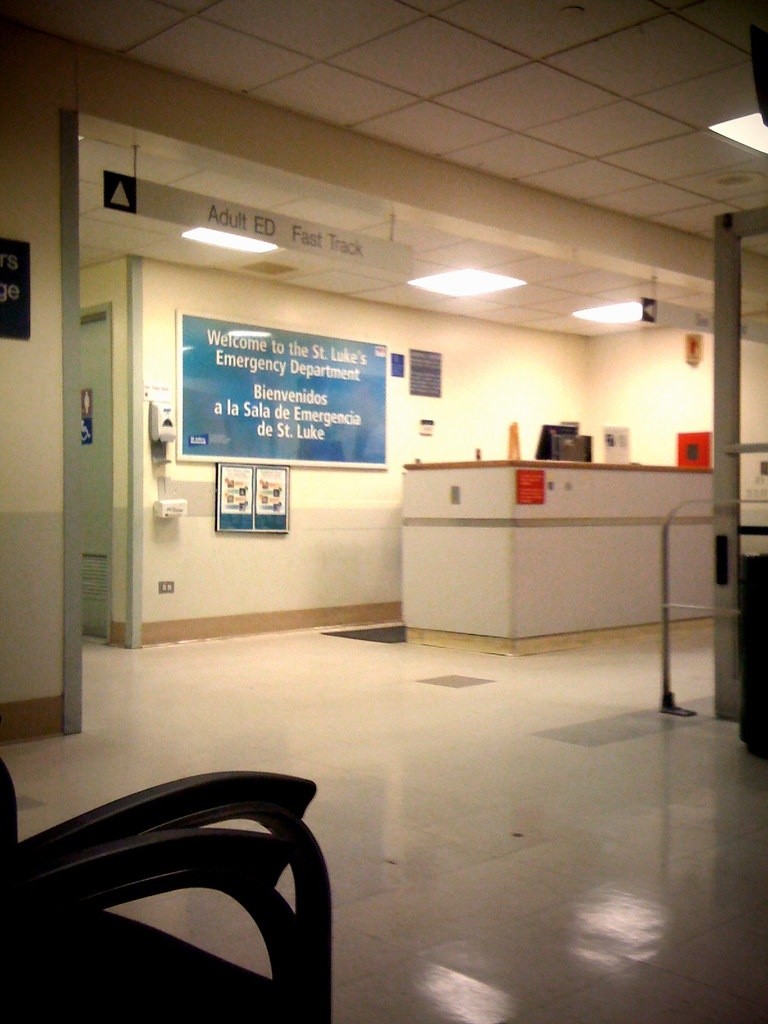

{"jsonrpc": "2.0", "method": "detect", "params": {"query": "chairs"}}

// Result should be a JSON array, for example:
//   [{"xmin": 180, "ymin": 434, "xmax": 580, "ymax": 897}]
[
  {"xmin": 0, "ymin": 760, "xmax": 333, "ymax": 971},
  {"xmin": 0, "ymin": 826, "xmax": 318, "ymax": 1024}
]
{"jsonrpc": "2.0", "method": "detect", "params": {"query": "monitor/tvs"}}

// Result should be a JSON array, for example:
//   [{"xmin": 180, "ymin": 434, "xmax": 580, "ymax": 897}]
[{"xmin": 535, "ymin": 425, "xmax": 577, "ymax": 460}]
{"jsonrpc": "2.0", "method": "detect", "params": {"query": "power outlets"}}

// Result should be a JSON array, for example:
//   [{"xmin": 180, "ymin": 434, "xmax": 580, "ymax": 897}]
[{"xmin": 158, "ymin": 581, "xmax": 174, "ymax": 594}]
